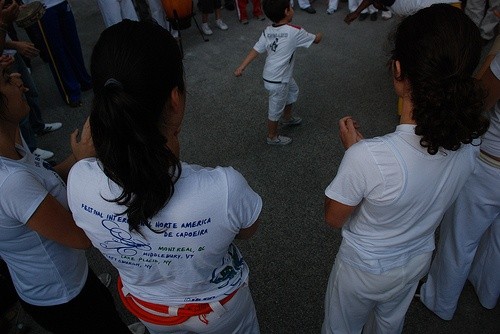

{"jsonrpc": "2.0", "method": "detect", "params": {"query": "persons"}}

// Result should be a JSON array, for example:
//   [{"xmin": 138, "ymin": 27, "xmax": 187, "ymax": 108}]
[
  {"xmin": 67, "ymin": 19, "xmax": 262, "ymax": 334},
  {"xmin": 0, "ymin": 0, "xmax": 500, "ymax": 159},
  {"xmin": 322, "ymin": 3, "xmax": 491, "ymax": 334},
  {"xmin": 415, "ymin": 39, "xmax": 500, "ymax": 320},
  {"xmin": 0, "ymin": 70, "xmax": 146, "ymax": 334}
]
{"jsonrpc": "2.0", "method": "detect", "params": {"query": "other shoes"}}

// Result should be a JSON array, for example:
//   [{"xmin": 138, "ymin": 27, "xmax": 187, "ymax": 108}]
[
  {"xmin": 215, "ymin": 19, "xmax": 229, "ymax": 31},
  {"xmin": 97, "ymin": 272, "xmax": 112, "ymax": 288},
  {"xmin": 128, "ymin": 322, "xmax": 146, "ymax": 334},
  {"xmin": 281, "ymin": 116, "xmax": 302, "ymax": 125},
  {"xmin": 32, "ymin": 147, "xmax": 55, "ymax": 160},
  {"xmin": 267, "ymin": 134, "xmax": 292, "ymax": 146},
  {"xmin": 327, "ymin": 9, "xmax": 338, "ymax": 15},
  {"xmin": 254, "ymin": 15, "xmax": 266, "ymax": 21},
  {"xmin": 38, "ymin": 121, "xmax": 63, "ymax": 136},
  {"xmin": 371, "ymin": 12, "xmax": 378, "ymax": 21},
  {"xmin": 48, "ymin": 161, "xmax": 56, "ymax": 167},
  {"xmin": 359, "ymin": 12, "xmax": 370, "ymax": 21},
  {"xmin": 240, "ymin": 18, "xmax": 249, "ymax": 25},
  {"xmin": 300, "ymin": 6, "xmax": 317, "ymax": 14},
  {"xmin": 201, "ymin": 22, "xmax": 213, "ymax": 36},
  {"xmin": 68, "ymin": 100, "xmax": 84, "ymax": 107},
  {"xmin": 81, "ymin": 82, "xmax": 93, "ymax": 91}
]
